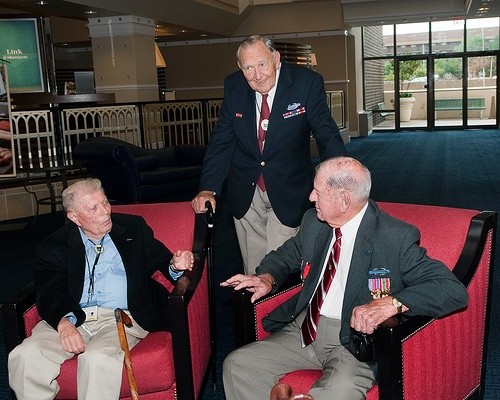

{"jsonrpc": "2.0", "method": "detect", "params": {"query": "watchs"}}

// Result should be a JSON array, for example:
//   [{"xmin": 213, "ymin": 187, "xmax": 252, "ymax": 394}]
[{"xmin": 392, "ymin": 297, "xmax": 402, "ymax": 313}]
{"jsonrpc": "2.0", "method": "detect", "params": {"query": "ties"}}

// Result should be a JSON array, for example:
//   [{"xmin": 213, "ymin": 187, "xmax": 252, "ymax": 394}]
[
  {"xmin": 256, "ymin": 94, "xmax": 272, "ymax": 193},
  {"xmin": 299, "ymin": 227, "xmax": 343, "ymax": 348}
]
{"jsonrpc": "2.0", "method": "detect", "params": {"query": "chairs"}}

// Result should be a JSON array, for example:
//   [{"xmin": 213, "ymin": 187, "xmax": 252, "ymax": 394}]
[
  {"xmin": 234, "ymin": 202, "xmax": 498, "ymax": 400},
  {"xmin": 0, "ymin": 202, "xmax": 211, "ymax": 400}
]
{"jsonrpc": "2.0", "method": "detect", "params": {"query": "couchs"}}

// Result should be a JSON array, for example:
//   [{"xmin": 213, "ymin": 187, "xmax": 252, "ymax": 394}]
[{"xmin": 72, "ymin": 137, "xmax": 208, "ymax": 204}]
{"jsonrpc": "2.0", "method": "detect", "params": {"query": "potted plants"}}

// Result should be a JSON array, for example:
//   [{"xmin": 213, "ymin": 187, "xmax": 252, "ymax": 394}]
[{"xmin": 384, "ymin": 52, "xmax": 424, "ymax": 122}]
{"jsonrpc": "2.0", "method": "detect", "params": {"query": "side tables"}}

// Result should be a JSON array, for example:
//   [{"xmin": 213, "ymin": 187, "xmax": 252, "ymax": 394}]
[{"xmin": 17, "ymin": 155, "xmax": 89, "ymax": 215}]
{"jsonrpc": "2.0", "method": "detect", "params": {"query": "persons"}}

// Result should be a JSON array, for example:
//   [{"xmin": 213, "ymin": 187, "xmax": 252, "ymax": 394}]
[
  {"xmin": 7, "ymin": 178, "xmax": 194, "ymax": 400},
  {"xmin": 190, "ymin": 34, "xmax": 348, "ymax": 350},
  {"xmin": 219, "ymin": 156, "xmax": 468, "ymax": 400}
]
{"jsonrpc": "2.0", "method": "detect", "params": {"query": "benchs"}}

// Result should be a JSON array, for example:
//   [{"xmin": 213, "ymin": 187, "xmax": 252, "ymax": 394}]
[
  {"xmin": 377, "ymin": 102, "xmax": 395, "ymax": 127},
  {"xmin": 434, "ymin": 98, "xmax": 486, "ymax": 120}
]
